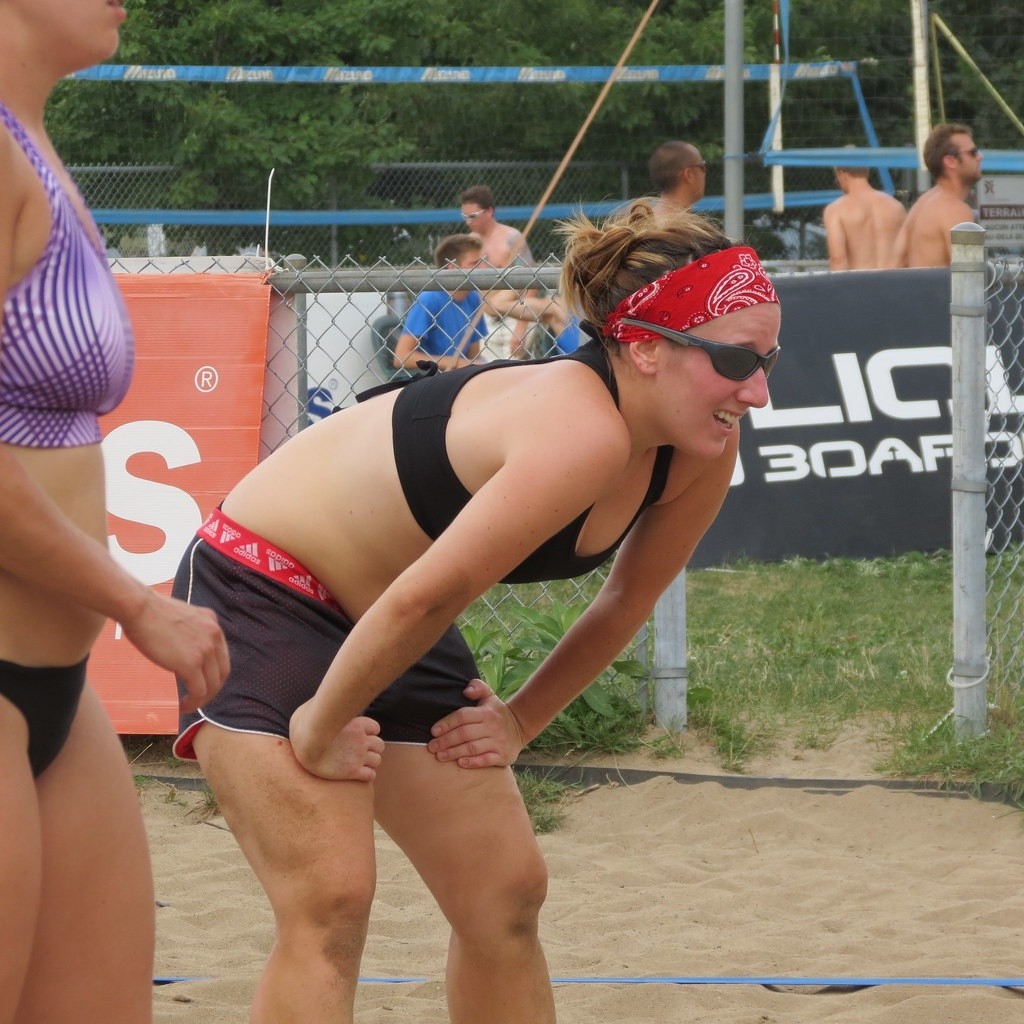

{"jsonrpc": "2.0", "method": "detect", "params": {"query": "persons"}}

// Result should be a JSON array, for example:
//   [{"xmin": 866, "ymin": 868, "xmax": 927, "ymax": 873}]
[
  {"xmin": 0, "ymin": 0, "xmax": 232, "ymax": 1024},
  {"xmin": 172, "ymin": 199, "xmax": 782, "ymax": 1024},
  {"xmin": 393, "ymin": 187, "xmax": 579, "ymax": 375},
  {"xmin": 823, "ymin": 144, "xmax": 908, "ymax": 270},
  {"xmin": 649, "ymin": 141, "xmax": 706, "ymax": 226},
  {"xmin": 892, "ymin": 122, "xmax": 982, "ymax": 270}
]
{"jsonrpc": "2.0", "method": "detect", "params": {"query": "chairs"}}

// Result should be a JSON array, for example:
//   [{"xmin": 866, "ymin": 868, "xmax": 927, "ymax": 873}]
[{"xmin": 374, "ymin": 317, "xmax": 436, "ymax": 382}]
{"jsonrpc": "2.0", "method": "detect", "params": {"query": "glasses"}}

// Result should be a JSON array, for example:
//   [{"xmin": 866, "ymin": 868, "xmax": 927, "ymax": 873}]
[
  {"xmin": 951, "ymin": 147, "xmax": 979, "ymax": 157},
  {"xmin": 622, "ymin": 317, "xmax": 780, "ymax": 380},
  {"xmin": 683, "ymin": 160, "xmax": 707, "ymax": 173},
  {"xmin": 462, "ymin": 208, "xmax": 486, "ymax": 220}
]
